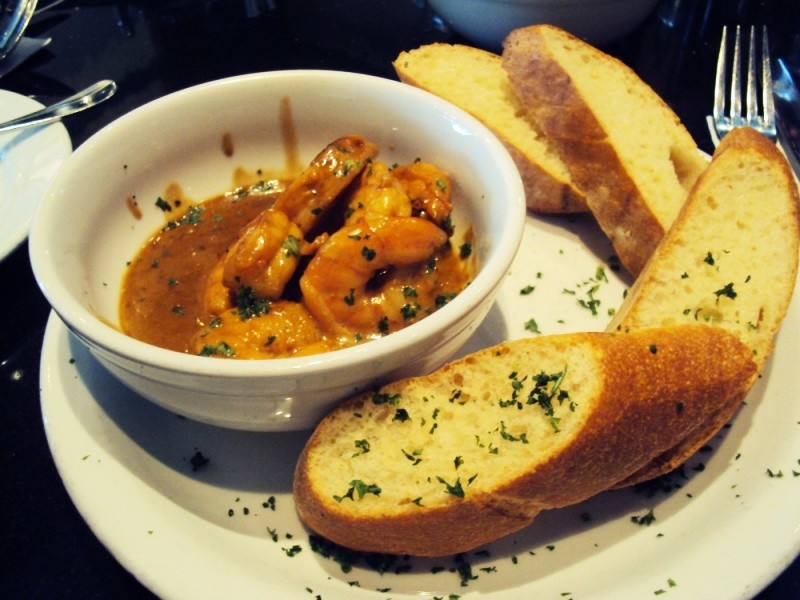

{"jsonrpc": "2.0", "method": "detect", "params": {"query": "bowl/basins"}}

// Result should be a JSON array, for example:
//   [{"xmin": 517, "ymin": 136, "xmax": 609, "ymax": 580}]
[{"xmin": 28, "ymin": 70, "xmax": 527, "ymax": 433}]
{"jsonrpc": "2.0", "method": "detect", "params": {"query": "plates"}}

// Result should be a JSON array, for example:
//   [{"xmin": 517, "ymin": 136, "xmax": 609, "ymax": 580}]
[
  {"xmin": 40, "ymin": 148, "xmax": 800, "ymax": 600},
  {"xmin": 0, "ymin": 89, "xmax": 72, "ymax": 263}
]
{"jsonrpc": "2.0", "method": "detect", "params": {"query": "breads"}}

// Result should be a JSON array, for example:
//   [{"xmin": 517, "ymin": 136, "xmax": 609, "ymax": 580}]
[
  {"xmin": 392, "ymin": 43, "xmax": 595, "ymax": 214},
  {"xmin": 602, "ymin": 127, "xmax": 800, "ymax": 493},
  {"xmin": 502, "ymin": 24, "xmax": 709, "ymax": 286},
  {"xmin": 290, "ymin": 326, "xmax": 756, "ymax": 553}
]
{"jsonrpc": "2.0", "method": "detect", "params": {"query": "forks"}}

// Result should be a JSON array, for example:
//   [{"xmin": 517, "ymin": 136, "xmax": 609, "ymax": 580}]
[{"xmin": 713, "ymin": 24, "xmax": 777, "ymax": 146}]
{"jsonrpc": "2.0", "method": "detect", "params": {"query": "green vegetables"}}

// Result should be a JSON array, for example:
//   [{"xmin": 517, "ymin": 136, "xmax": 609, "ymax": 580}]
[{"xmin": 127, "ymin": 141, "xmax": 799, "ymax": 600}]
